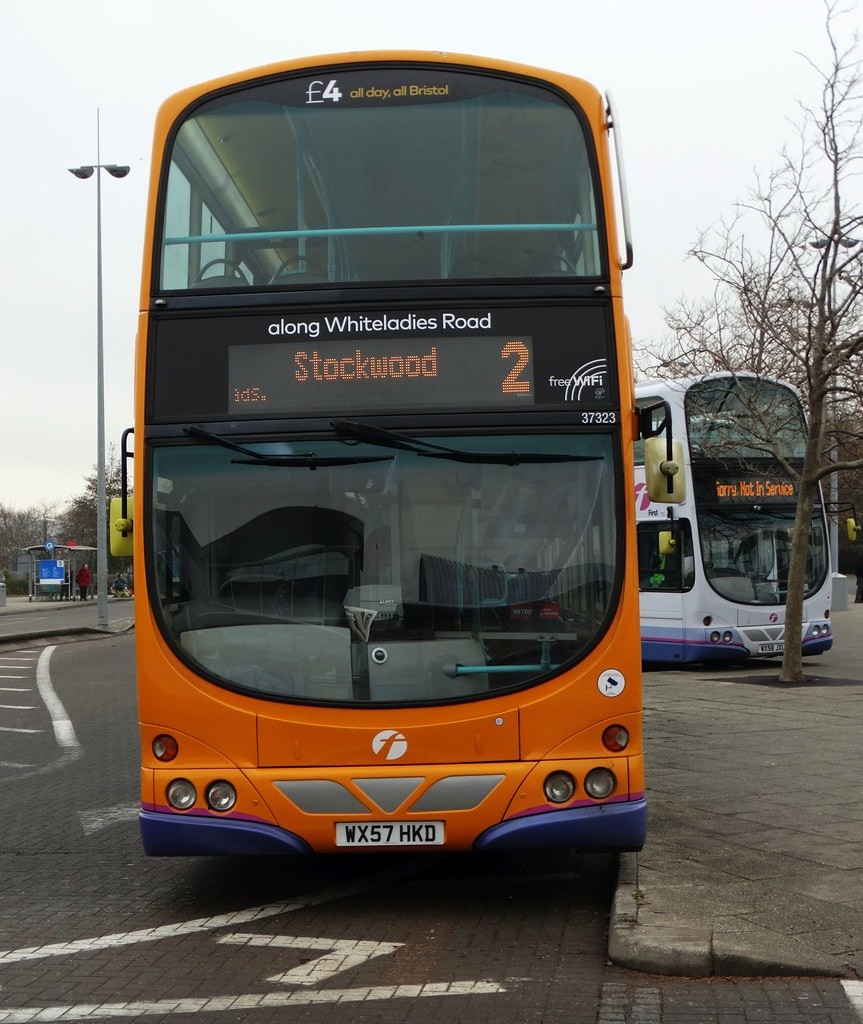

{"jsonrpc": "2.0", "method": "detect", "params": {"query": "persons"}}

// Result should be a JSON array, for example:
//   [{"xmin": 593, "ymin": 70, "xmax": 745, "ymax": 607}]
[
  {"xmin": 76, "ymin": 563, "xmax": 92, "ymax": 601},
  {"xmin": 48, "ymin": 564, "xmax": 74, "ymax": 601},
  {"xmin": 110, "ymin": 572, "xmax": 128, "ymax": 596}
]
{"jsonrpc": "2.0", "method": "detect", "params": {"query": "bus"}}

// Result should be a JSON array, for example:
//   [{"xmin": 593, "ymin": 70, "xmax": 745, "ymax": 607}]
[
  {"xmin": 109, "ymin": 50, "xmax": 686, "ymax": 859},
  {"xmin": 633, "ymin": 371, "xmax": 861, "ymax": 664}
]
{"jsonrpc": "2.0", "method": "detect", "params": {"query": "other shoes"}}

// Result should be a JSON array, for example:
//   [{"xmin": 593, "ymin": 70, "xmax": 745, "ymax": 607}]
[{"xmin": 79, "ymin": 596, "xmax": 87, "ymax": 601}]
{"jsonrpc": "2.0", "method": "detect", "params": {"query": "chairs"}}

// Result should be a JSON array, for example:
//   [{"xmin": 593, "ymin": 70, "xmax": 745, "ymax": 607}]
[
  {"xmin": 445, "ymin": 254, "xmax": 498, "ymax": 278},
  {"xmin": 522, "ymin": 253, "xmax": 579, "ymax": 276},
  {"xmin": 267, "ymin": 255, "xmax": 329, "ymax": 284},
  {"xmin": 187, "ymin": 258, "xmax": 250, "ymax": 288}
]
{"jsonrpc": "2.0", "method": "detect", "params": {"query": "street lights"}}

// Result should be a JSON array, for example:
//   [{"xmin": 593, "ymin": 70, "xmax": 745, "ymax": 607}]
[{"xmin": 67, "ymin": 164, "xmax": 131, "ymax": 630}]
